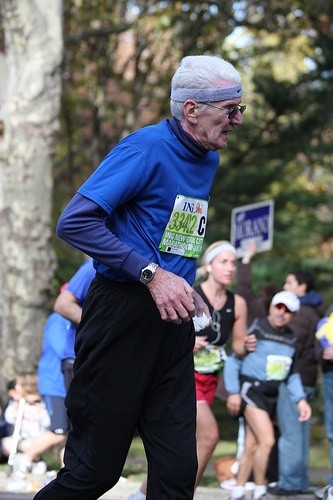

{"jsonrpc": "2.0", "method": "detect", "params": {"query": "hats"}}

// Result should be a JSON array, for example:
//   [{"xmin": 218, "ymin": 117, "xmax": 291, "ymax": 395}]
[{"xmin": 272, "ymin": 291, "xmax": 300, "ymax": 311}]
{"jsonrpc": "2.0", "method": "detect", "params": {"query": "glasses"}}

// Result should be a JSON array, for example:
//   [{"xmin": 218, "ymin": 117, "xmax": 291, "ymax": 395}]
[{"xmin": 203, "ymin": 101, "xmax": 247, "ymax": 119}]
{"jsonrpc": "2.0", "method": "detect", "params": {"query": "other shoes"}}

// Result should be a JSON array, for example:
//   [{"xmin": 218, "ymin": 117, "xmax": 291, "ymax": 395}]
[
  {"xmin": 221, "ymin": 478, "xmax": 237, "ymax": 491},
  {"xmin": 228, "ymin": 493, "xmax": 244, "ymax": 500},
  {"xmin": 128, "ymin": 491, "xmax": 146, "ymax": 500},
  {"xmin": 269, "ymin": 481, "xmax": 300, "ymax": 494},
  {"xmin": 250, "ymin": 490, "xmax": 269, "ymax": 500},
  {"xmin": 315, "ymin": 484, "xmax": 333, "ymax": 500}
]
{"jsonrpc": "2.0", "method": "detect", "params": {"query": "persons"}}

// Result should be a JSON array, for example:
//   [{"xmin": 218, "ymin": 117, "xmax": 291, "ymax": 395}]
[
  {"xmin": 0, "ymin": 258, "xmax": 97, "ymax": 483},
  {"xmin": 220, "ymin": 269, "xmax": 333, "ymax": 500},
  {"xmin": 223, "ymin": 291, "xmax": 313, "ymax": 500},
  {"xmin": 128, "ymin": 241, "xmax": 257, "ymax": 500},
  {"xmin": 32, "ymin": 55, "xmax": 247, "ymax": 500}
]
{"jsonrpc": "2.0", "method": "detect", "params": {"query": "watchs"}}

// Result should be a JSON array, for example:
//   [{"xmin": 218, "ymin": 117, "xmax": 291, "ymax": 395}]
[{"xmin": 139, "ymin": 262, "xmax": 159, "ymax": 284}]
